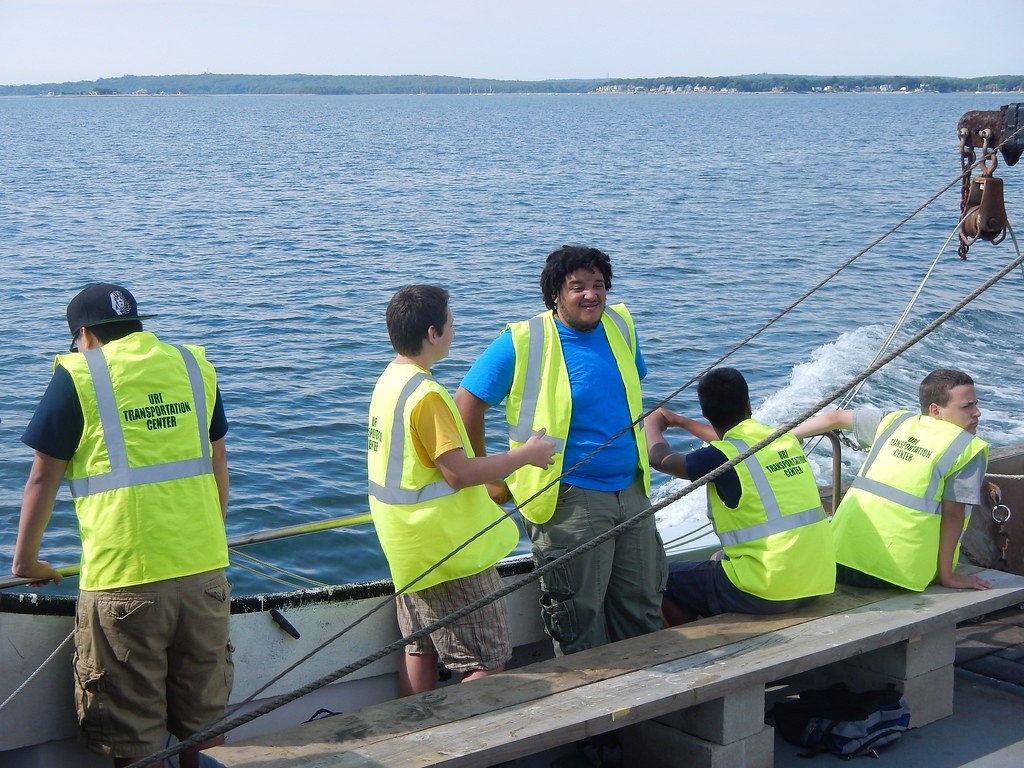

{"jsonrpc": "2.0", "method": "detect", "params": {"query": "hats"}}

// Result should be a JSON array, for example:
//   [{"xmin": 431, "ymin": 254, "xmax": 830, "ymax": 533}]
[{"xmin": 66, "ymin": 282, "xmax": 157, "ymax": 335}]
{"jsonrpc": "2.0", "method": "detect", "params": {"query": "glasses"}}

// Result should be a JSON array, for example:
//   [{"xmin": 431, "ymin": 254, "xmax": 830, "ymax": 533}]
[{"xmin": 69, "ymin": 331, "xmax": 80, "ymax": 352}]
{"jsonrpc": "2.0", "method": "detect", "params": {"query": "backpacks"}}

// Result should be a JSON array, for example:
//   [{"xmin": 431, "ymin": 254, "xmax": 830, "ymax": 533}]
[{"xmin": 774, "ymin": 680, "xmax": 910, "ymax": 761}]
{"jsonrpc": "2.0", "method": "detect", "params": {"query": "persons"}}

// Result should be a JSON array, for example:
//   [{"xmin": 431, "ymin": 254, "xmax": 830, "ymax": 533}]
[
  {"xmin": 452, "ymin": 245, "xmax": 669, "ymax": 658},
  {"xmin": 366, "ymin": 284, "xmax": 558, "ymax": 700},
  {"xmin": 779, "ymin": 366, "xmax": 992, "ymax": 592},
  {"xmin": 644, "ymin": 366, "xmax": 837, "ymax": 627},
  {"xmin": 12, "ymin": 281, "xmax": 234, "ymax": 768}
]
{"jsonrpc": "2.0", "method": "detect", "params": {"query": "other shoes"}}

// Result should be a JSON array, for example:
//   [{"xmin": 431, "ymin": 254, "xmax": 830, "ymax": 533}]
[{"xmin": 575, "ymin": 731, "xmax": 624, "ymax": 768}]
